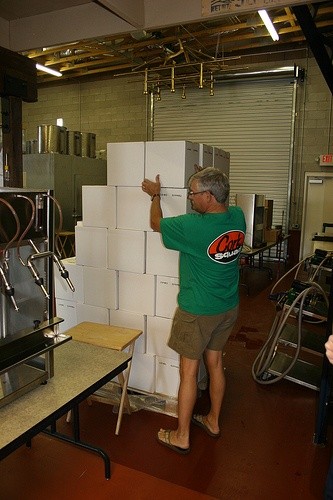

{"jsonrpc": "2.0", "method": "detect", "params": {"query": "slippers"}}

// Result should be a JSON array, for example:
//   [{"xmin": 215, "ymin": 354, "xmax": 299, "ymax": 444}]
[
  {"xmin": 191, "ymin": 415, "xmax": 220, "ymax": 437},
  {"xmin": 156, "ymin": 429, "xmax": 192, "ymax": 454}
]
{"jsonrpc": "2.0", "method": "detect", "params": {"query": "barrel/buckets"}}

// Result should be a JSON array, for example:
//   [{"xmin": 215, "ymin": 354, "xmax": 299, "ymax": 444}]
[
  {"xmin": 81, "ymin": 133, "xmax": 96, "ymax": 158},
  {"xmin": 36, "ymin": 124, "xmax": 67, "ymax": 154},
  {"xmin": 67, "ymin": 131, "xmax": 81, "ymax": 156}
]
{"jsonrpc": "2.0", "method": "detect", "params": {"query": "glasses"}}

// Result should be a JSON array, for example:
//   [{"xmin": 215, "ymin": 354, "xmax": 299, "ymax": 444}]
[{"xmin": 189, "ymin": 190, "xmax": 207, "ymax": 196}]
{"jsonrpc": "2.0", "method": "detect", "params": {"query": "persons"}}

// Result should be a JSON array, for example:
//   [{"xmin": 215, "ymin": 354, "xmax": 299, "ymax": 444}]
[{"xmin": 141, "ymin": 167, "xmax": 246, "ymax": 453}]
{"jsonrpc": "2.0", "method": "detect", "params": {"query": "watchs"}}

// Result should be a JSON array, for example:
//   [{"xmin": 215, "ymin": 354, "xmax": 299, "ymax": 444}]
[{"xmin": 151, "ymin": 194, "xmax": 160, "ymax": 201}]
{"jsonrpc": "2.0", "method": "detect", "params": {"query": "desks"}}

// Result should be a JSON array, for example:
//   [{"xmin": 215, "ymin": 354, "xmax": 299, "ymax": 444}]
[
  {"xmin": 55, "ymin": 231, "xmax": 75, "ymax": 258},
  {"xmin": 0, "ymin": 339, "xmax": 133, "ymax": 480},
  {"xmin": 239, "ymin": 233, "xmax": 292, "ymax": 296},
  {"xmin": 63, "ymin": 322, "xmax": 142, "ymax": 436}
]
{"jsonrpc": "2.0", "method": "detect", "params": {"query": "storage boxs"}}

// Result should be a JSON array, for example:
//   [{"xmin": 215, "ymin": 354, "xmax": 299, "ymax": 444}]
[
  {"xmin": 145, "ymin": 140, "xmax": 199, "ymax": 189},
  {"xmin": 212, "ymin": 147, "xmax": 230, "ymax": 179},
  {"xmin": 198, "ymin": 143, "xmax": 213, "ymax": 168},
  {"xmin": 54, "ymin": 186, "xmax": 208, "ymax": 399},
  {"xmin": 107, "ymin": 142, "xmax": 145, "ymax": 187},
  {"xmin": 264, "ymin": 230, "xmax": 280, "ymax": 242}
]
